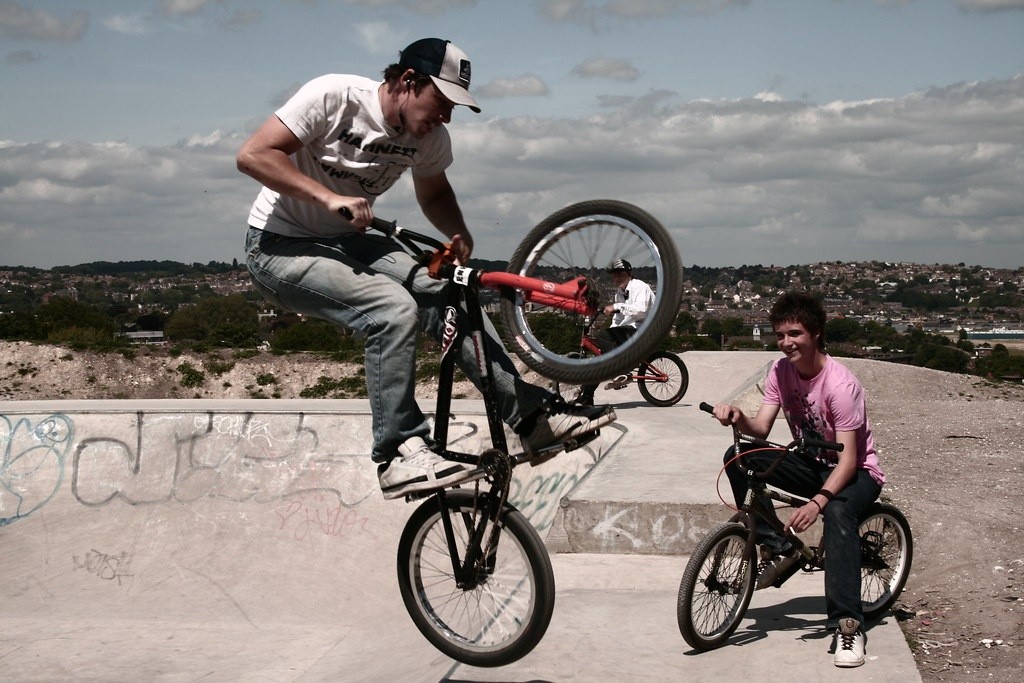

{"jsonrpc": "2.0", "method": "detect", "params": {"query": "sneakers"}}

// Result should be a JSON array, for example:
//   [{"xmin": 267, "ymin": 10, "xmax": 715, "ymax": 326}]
[
  {"xmin": 754, "ymin": 544, "xmax": 801, "ymax": 589},
  {"xmin": 377, "ymin": 435, "xmax": 469, "ymax": 500},
  {"xmin": 833, "ymin": 618, "xmax": 865, "ymax": 667},
  {"xmin": 520, "ymin": 405, "xmax": 616, "ymax": 466}
]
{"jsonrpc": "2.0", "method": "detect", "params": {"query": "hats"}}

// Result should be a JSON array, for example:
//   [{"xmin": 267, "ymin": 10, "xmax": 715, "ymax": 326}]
[{"xmin": 400, "ymin": 38, "xmax": 482, "ymax": 113}]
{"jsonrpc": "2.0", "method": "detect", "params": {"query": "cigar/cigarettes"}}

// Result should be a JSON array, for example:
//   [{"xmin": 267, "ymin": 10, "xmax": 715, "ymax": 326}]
[{"xmin": 789, "ymin": 525, "xmax": 797, "ymax": 537}]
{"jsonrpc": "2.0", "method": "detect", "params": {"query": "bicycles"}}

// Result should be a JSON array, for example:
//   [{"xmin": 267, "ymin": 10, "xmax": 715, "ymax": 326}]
[
  {"xmin": 553, "ymin": 314, "xmax": 691, "ymax": 407},
  {"xmin": 676, "ymin": 400, "xmax": 916, "ymax": 651},
  {"xmin": 340, "ymin": 200, "xmax": 686, "ymax": 666}
]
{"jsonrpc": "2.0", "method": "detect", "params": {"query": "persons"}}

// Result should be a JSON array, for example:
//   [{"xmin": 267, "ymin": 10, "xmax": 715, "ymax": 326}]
[
  {"xmin": 568, "ymin": 258, "xmax": 659, "ymax": 406},
  {"xmin": 237, "ymin": 38, "xmax": 617, "ymax": 501},
  {"xmin": 712, "ymin": 293, "xmax": 885, "ymax": 669}
]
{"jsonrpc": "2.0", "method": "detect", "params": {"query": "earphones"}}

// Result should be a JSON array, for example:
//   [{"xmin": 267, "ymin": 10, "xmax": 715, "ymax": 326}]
[{"xmin": 405, "ymin": 77, "xmax": 412, "ymax": 93}]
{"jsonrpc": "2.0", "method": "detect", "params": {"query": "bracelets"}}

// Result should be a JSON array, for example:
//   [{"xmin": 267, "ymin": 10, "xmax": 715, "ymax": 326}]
[{"xmin": 810, "ymin": 499, "xmax": 822, "ymax": 512}]
